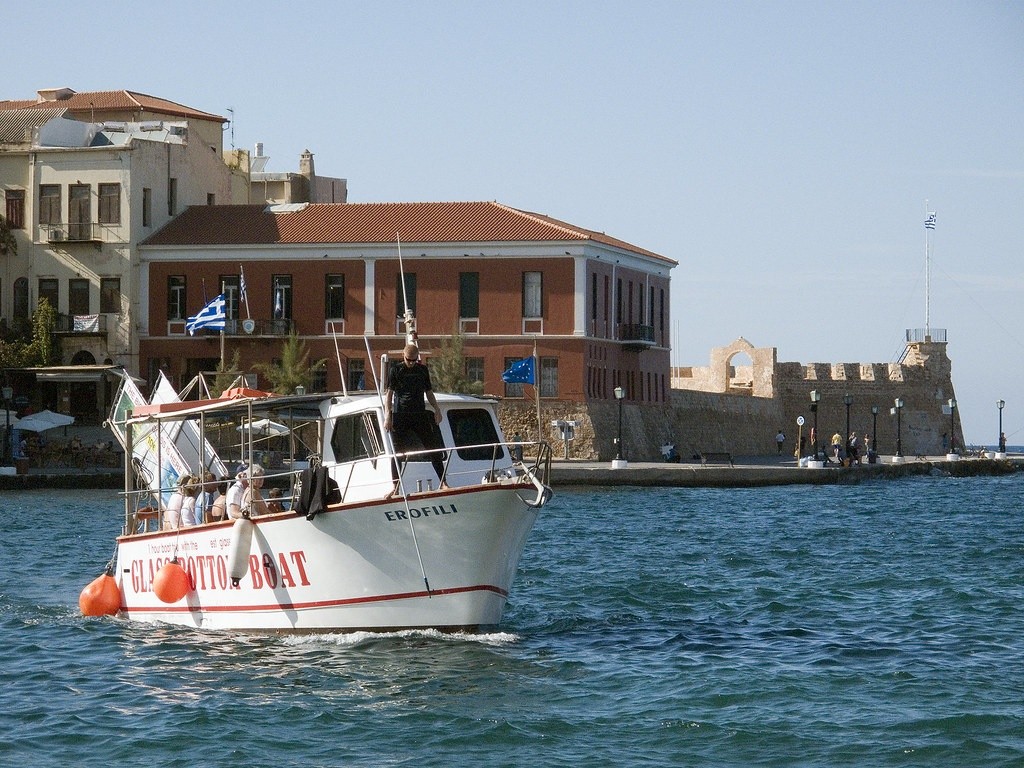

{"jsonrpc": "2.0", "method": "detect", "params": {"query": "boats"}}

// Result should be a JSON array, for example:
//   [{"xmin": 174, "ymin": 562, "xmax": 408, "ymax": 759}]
[{"xmin": 114, "ymin": 230, "xmax": 554, "ymax": 637}]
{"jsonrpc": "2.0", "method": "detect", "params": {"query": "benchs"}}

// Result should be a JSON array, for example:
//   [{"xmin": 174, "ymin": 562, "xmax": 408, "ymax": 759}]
[
  {"xmin": 915, "ymin": 450, "xmax": 927, "ymax": 461},
  {"xmin": 702, "ymin": 453, "xmax": 735, "ymax": 468}
]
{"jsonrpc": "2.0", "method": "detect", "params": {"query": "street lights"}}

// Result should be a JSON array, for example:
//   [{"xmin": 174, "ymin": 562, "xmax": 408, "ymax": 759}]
[
  {"xmin": 948, "ymin": 398, "xmax": 958, "ymax": 454},
  {"xmin": 2, "ymin": 383, "xmax": 13, "ymax": 429},
  {"xmin": 872, "ymin": 404, "xmax": 880, "ymax": 458},
  {"xmin": 894, "ymin": 397, "xmax": 905, "ymax": 457},
  {"xmin": 614, "ymin": 386, "xmax": 626, "ymax": 460},
  {"xmin": 843, "ymin": 392, "xmax": 853, "ymax": 454},
  {"xmin": 810, "ymin": 389, "xmax": 821, "ymax": 461},
  {"xmin": 996, "ymin": 399, "xmax": 1005, "ymax": 453}
]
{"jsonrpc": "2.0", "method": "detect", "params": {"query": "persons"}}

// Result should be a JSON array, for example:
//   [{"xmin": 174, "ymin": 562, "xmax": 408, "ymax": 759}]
[
  {"xmin": 776, "ymin": 430, "xmax": 786, "ymax": 456},
  {"xmin": 164, "ymin": 464, "xmax": 285, "ymax": 529},
  {"xmin": 510, "ymin": 432, "xmax": 523, "ymax": 460},
  {"xmin": 669, "ymin": 446, "xmax": 681, "ymax": 463},
  {"xmin": 831, "ymin": 430, "xmax": 871, "ymax": 459},
  {"xmin": 19, "ymin": 435, "xmax": 113, "ymax": 457},
  {"xmin": 998, "ymin": 432, "xmax": 1007, "ymax": 453},
  {"xmin": 384, "ymin": 344, "xmax": 451, "ymax": 499},
  {"xmin": 943, "ymin": 432, "xmax": 956, "ymax": 454}
]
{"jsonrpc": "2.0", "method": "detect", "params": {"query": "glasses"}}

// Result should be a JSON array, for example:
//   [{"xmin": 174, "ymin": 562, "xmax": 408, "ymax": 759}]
[{"xmin": 405, "ymin": 356, "xmax": 418, "ymax": 361}]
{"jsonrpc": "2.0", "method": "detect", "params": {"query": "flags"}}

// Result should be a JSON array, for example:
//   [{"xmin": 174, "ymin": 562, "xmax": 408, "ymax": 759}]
[
  {"xmin": 501, "ymin": 355, "xmax": 537, "ymax": 385},
  {"xmin": 924, "ymin": 210, "xmax": 937, "ymax": 230},
  {"xmin": 185, "ymin": 292, "xmax": 226, "ymax": 337},
  {"xmin": 239, "ymin": 266, "xmax": 247, "ymax": 303},
  {"xmin": 274, "ymin": 283, "xmax": 283, "ymax": 318}
]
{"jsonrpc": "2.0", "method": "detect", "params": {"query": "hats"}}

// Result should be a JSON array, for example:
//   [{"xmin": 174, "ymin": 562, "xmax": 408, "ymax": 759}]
[
  {"xmin": 236, "ymin": 464, "xmax": 248, "ymax": 472},
  {"xmin": 177, "ymin": 475, "xmax": 191, "ymax": 486}
]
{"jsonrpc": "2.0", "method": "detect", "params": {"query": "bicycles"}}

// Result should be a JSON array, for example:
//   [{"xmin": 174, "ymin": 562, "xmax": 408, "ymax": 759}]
[
  {"xmin": 40, "ymin": 444, "xmax": 118, "ymax": 470},
  {"xmin": 964, "ymin": 441, "xmax": 989, "ymax": 459}
]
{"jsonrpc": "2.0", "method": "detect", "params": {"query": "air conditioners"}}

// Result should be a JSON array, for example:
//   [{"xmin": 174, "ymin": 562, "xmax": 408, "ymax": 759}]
[
  {"xmin": 170, "ymin": 126, "xmax": 184, "ymax": 135},
  {"xmin": 50, "ymin": 228, "xmax": 63, "ymax": 242},
  {"xmin": 139, "ymin": 121, "xmax": 163, "ymax": 132},
  {"xmin": 104, "ymin": 120, "xmax": 128, "ymax": 133}
]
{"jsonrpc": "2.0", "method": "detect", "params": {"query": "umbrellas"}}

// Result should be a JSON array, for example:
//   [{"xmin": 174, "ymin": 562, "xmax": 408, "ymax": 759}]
[{"xmin": 236, "ymin": 419, "xmax": 291, "ymax": 448}]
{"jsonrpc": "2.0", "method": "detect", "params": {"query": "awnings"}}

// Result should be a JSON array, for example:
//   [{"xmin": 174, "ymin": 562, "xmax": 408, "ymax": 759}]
[{"xmin": 107, "ymin": 368, "xmax": 147, "ymax": 386}]
